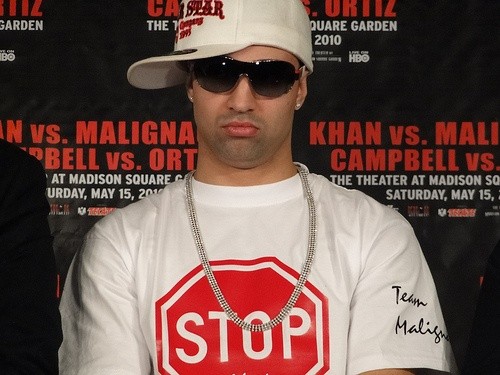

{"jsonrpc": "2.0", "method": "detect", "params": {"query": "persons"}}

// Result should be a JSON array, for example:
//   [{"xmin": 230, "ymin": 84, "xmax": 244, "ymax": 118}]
[{"xmin": 58, "ymin": 0, "xmax": 459, "ymax": 375}]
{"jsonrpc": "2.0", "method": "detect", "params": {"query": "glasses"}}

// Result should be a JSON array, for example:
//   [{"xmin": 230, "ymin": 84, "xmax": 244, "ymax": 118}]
[{"xmin": 189, "ymin": 55, "xmax": 306, "ymax": 97}]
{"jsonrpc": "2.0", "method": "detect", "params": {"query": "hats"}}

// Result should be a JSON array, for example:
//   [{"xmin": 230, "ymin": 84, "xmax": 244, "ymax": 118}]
[{"xmin": 127, "ymin": 0, "xmax": 313, "ymax": 89}]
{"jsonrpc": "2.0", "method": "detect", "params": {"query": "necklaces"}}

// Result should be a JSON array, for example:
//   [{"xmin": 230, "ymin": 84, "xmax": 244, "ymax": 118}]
[{"xmin": 185, "ymin": 164, "xmax": 317, "ymax": 332}]
